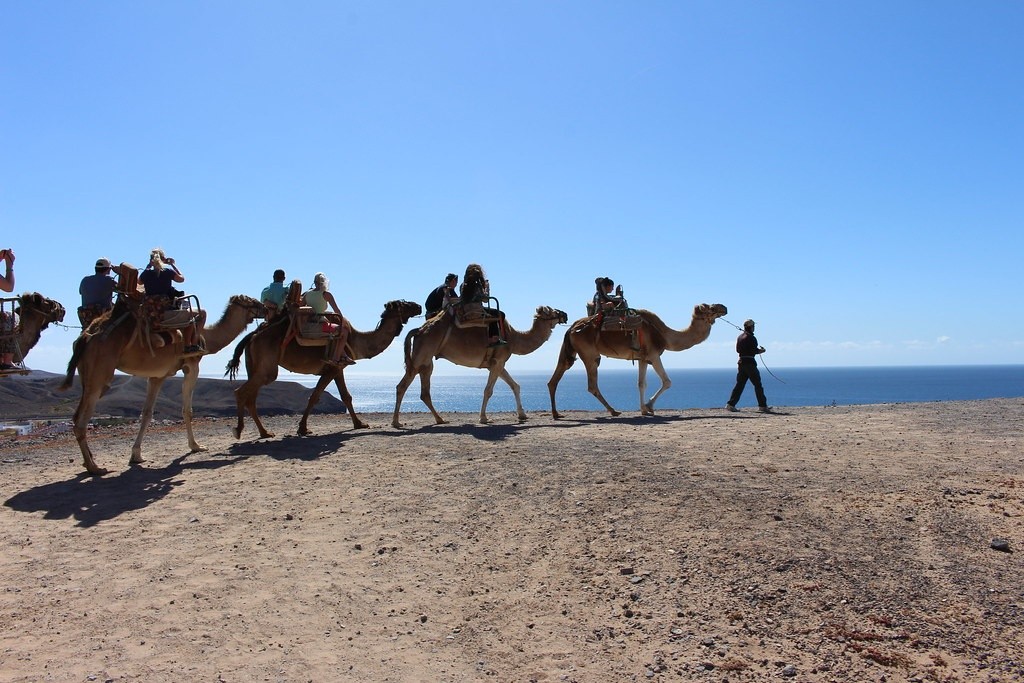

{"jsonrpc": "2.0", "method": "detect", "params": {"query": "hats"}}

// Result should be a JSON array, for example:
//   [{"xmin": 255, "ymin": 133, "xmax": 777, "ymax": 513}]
[
  {"xmin": 744, "ymin": 318, "xmax": 755, "ymax": 326},
  {"xmin": 95, "ymin": 257, "xmax": 112, "ymax": 267}
]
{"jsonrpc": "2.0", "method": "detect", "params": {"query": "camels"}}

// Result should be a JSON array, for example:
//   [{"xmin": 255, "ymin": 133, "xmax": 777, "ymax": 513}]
[
  {"xmin": 224, "ymin": 299, "xmax": 422, "ymax": 440},
  {"xmin": 391, "ymin": 302, "xmax": 568, "ymax": 428},
  {"xmin": 547, "ymin": 303, "xmax": 727, "ymax": 419},
  {"xmin": 57, "ymin": 295, "xmax": 269, "ymax": 476},
  {"xmin": 0, "ymin": 291, "xmax": 66, "ymax": 377}
]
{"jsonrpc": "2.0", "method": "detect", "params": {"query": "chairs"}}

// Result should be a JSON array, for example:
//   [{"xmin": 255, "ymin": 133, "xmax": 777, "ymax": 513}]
[
  {"xmin": 116, "ymin": 264, "xmax": 201, "ymax": 328},
  {"xmin": 262, "ymin": 299, "xmax": 278, "ymax": 321},
  {"xmin": 289, "ymin": 283, "xmax": 342, "ymax": 340},
  {"xmin": 598, "ymin": 309, "xmax": 636, "ymax": 330},
  {"xmin": 447, "ymin": 296, "xmax": 500, "ymax": 324},
  {"xmin": 76, "ymin": 303, "xmax": 111, "ymax": 326}
]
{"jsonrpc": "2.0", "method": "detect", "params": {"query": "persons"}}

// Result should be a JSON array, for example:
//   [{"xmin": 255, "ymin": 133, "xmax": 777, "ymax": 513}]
[
  {"xmin": 298, "ymin": 272, "xmax": 357, "ymax": 365},
  {"xmin": 79, "ymin": 256, "xmax": 121, "ymax": 309},
  {"xmin": 459, "ymin": 264, "xmax": 507, "ymax": 346},
  {"xmin": 725, "ymin": 319, "xmax": 773, "ymax": 412},
  {"xmin": 593, "ymin": 277, "xmax": 628, "ymax": 322},
  {"xmin": 0, "ymin": 247, "xmax": 21, "ymax": 370},
  {"xmin": 425, "ymin": 273, "xmax": 459, "ymax": 321},
  {"xmin": 138, "ymin": 248, "xmax": 207, "ymax": 354},
  {"xmin": 260, "ymin": 269, "xmax": 289, "ymax": 309}
]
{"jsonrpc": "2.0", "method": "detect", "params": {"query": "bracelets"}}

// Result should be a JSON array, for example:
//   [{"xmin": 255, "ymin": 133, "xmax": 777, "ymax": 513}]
[{"xmin": 6, "ymin": 268, "xmax": 13, "ymax": 271}]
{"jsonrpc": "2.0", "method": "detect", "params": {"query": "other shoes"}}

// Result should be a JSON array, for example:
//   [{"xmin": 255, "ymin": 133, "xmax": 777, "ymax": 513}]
[
  {"xmin": 488, "ymin": 338, "xmax": 508, "ymax": 345},
  {"xmin": 725, "ymin": 404, "xmax": 740, "ymax": 412},
  {"xmin": 758, "ymin": 405, "xmax": 773, "ymax": 411},
  {"xmin": 183, "ymin": 345, "xmax": 192, "ymax": 353},
  {"xmin": 1, "ymin": 362, "xmax": 22, "ymax": 370},
  {"xmin": 190, "ymin": 344, "xmax": 205, "ymax": 353}
]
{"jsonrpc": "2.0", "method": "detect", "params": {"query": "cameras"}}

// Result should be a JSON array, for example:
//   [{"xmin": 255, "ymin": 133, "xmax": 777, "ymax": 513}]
[
  {"xmin": 165, "ymin": 259, "xmax": 168, "ymax": 262},
  {"xmin": 2, "ymin": 250, "xmax": 7, "ymax": 258}
]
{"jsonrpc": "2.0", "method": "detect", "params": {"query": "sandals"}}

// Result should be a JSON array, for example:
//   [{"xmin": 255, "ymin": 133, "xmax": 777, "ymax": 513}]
[{"xmin": 340, "ymin": 355, "xmax": 356, "ymax": 365}]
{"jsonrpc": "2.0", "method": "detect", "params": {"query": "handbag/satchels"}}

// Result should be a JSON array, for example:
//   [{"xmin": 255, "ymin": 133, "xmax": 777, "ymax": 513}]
[{"xmin": 296, "ymin": 306, "xmax": 323, "ymax": 339}]
